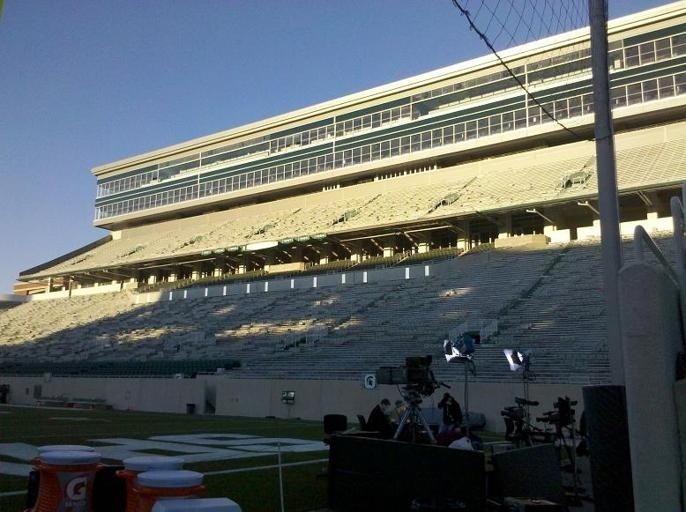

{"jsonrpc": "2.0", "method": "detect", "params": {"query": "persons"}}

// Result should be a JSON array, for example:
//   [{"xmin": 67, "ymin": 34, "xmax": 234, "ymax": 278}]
[
  {"xmin": 438, "ymin": 392, "xmax": 462, "ymax": 434},
  {"xmin": 366, "ymin": 399, "xmax": 391, "ymax": 431},
  {"xmin": 392, "ymin": 400, "xmax": 408, "ymax": 421}
]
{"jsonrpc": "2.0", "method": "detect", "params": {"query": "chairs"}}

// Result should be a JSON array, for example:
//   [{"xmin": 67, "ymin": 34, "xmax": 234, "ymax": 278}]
[
  {"xmin": 356, "ymin": 414, "xmax": 367, "ymax": 431},
  {"xmin": 323, "ymin": 414, "xmax": 348, "ymax": 444},
  {"xmin": 492, "ymin": 443, "xmax": 571, "ymax": 512}
]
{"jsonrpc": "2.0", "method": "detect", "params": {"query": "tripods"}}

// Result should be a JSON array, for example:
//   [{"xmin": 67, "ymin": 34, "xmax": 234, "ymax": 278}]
[{"xmin": 393, "ymin": 404, "xmax": 437, "ymax": 445}]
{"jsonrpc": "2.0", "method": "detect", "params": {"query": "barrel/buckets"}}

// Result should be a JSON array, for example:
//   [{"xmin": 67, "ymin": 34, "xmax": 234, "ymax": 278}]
[
  {"xmin": 36, "ymin": 444, "xmax": 96, "ymax": 456},
  {"xmin": 133, "ymin": 469, "xmax": 206, "ymax": 512},
  {"xmin": 31, "ymin": 450, "xmax": 102, "ymax": 512},
  {"xmin": 115, "ymin": 454, "xmax": 186, "ymax": 512}
]
